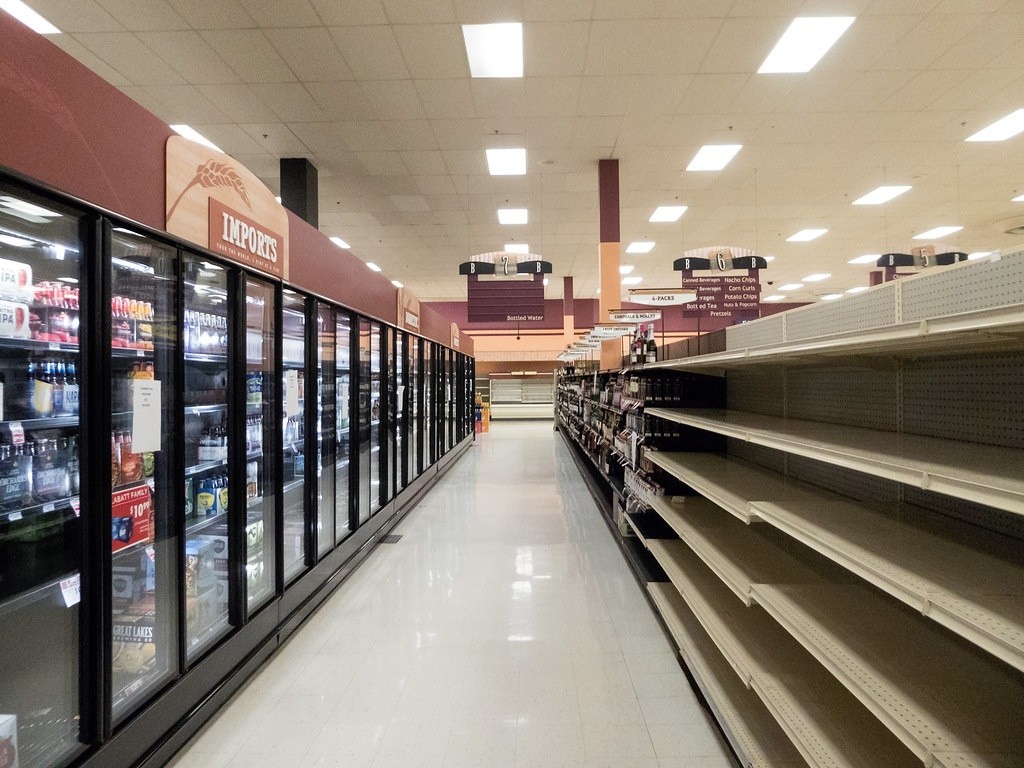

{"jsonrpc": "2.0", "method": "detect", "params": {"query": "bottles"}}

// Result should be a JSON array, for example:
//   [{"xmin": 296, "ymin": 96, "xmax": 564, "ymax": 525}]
[
  {"xmin": 34, "ymin": 280, "xmax": 79, "ymax": 310},
  {"xmin": 183, "ymin": 308, "xmax": 227, "ymax": 355},
  {"xmin": 0, "ymin": 435, "xmax": 80, "ymax": 511},
  {"xmin": 630, "ymin": 323, "xmax": 658, "ymax": 364},
  {"xmin": 26, "ymin": 356, "xmax": 79, "ymax": 418},
  {"xmin": 246, "ymin": 414, "xmax": 263, "ymax": 451},
  {"xmin": 111, "ymin": 430, "xmax": 132, "ymax": 445},
  {"xmin": 196, "ymin": 473, "xmax": 228, "ymax": 489},
  {"xmin": 111, "ymin": 296, "xmax": 154, "ymax": 321},
  {"xmin": 199, "ymin": 421, "xmax": 227, "ymax": 447}
]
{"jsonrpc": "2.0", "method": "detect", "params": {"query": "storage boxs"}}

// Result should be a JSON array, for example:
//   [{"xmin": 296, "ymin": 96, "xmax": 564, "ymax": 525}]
[{"xmin": 613, "ymin": 492, "xmax": 638, "ymax": 538}]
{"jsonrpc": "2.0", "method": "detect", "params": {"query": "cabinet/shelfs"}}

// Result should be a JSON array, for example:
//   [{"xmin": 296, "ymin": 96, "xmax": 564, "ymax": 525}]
[{"xmin": 553, "ymin": 244, "xmax": 1023, "ymax": 768}]
